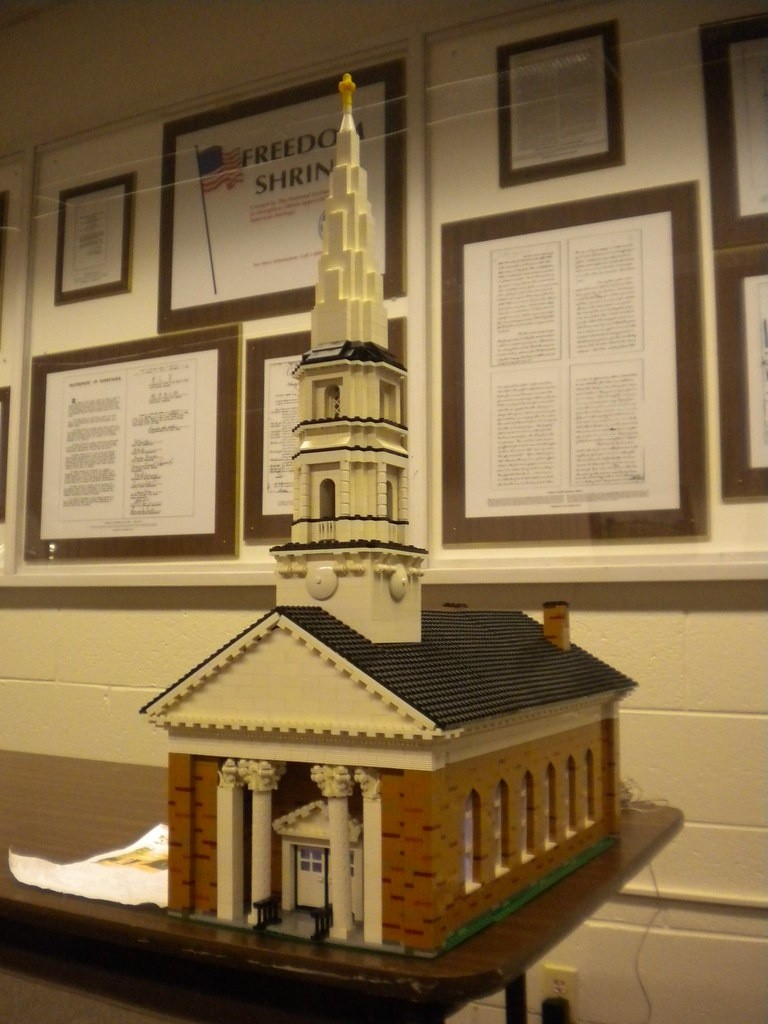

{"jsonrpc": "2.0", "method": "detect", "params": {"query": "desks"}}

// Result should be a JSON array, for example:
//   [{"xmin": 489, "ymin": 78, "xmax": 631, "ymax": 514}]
[{"xmin": 0, "ymin": 751, "xmax": 684, "ymax": 1024}]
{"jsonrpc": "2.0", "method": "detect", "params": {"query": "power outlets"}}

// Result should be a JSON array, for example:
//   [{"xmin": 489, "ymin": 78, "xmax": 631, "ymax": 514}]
[{"xmin": 542, "ymin": 963, "xmax": 578, "ymax": 1024}]
